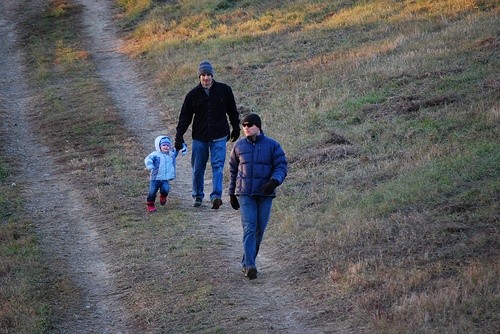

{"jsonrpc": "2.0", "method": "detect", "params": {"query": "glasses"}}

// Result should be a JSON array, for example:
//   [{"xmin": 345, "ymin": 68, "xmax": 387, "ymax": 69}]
[{"xmin": 242, "ymin": 123, "xmax": 253, "ymax": 127}]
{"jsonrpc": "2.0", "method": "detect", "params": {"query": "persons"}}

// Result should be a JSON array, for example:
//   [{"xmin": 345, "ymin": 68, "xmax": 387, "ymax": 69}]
[
  {"xmin": 175, "ymin": 61, "xmax": 241, "ymax": 209},
  {"xmin": 144, "ymin": 135, "xmax": 179, "ymax": 211},
  {"xmin": 228, "ymin": 114, "xmax": 288, "ymax": 280}
]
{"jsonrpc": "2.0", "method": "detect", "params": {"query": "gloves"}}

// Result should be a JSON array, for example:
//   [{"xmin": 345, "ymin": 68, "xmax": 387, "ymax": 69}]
[
  {"xmin": 175, "ymin": 138, "xmax": 184, "ymax": 150},
  {"xmin": 231, "ymin": 123, "xmax": 240, "ymax": 142},
  {"xmin": 262, "ymin": 181, "xmax": 278, "ymax": 194},
  {"xmin": 229, "ymin": 195, "xmax": 240, "ymax": 210}
]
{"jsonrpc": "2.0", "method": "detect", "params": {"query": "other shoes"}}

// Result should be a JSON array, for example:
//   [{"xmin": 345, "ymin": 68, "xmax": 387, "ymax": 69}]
[
  {"xmin": 159, "ymin": 194, "xmax": 166, "ymax": 205},
  {"xmin": 146, "ymin": 202, "xmax": 156, "ymax": 211},
  {"xmin": 192, "ymin": 198, "xmax": 202, "ymax": 206},
  {"xmin": 246, "ymin": 266, "xmax": 257, "ymax": 280},
  {"xmin": 211, "ymin": 197, "xmax": 222, "ymax": 209}
]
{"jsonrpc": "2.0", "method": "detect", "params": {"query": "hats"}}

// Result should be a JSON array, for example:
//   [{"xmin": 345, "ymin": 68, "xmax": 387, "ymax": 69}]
[
  {"xmin": 244, "ymin": 114, "xmax": 261, "ymax": 129},
  {"xmin": 198, "ymin": 61, "xmax": 213, "ymax": 77},
  {"xmin": 159, "ymin": 138, "xmax": 171, "ymax": 147}
]
